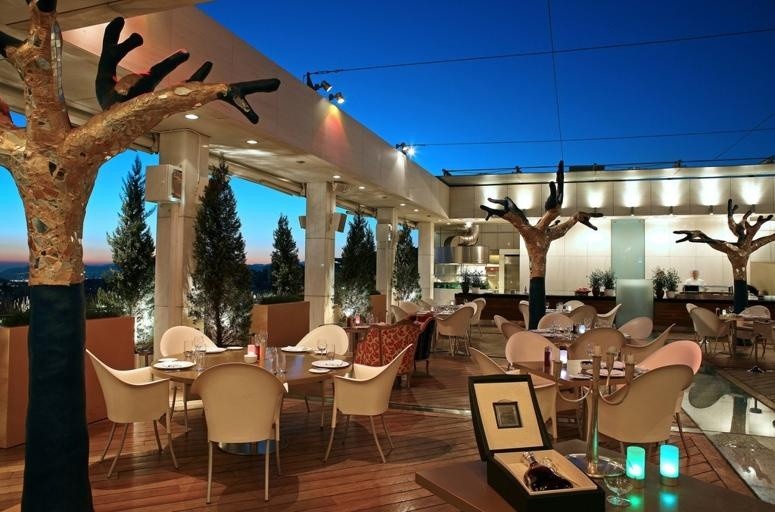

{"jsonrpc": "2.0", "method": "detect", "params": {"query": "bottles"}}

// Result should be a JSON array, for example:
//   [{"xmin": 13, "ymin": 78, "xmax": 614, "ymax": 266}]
[
  {"xmin": 347, "ymin": 317, "xmax": 351, "ymax": 327},
  {"xmin": 544, "ymin": 345, "xmax": 552, "ymax": 367},
  {"xmin": 519, "ymin": 451, "xmax": 573, "ymax": 492},
  {"xmin": 716, "ymin": 308, "xmax": 721, "ymax": 317},
  {"xmin": 255, "ymin": 343, "xmax": 260, "ymax": 360}
]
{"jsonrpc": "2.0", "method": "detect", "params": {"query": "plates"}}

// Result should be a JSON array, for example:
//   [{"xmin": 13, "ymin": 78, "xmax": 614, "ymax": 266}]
[
  {"xmin": 315, "ymin": 351, "xmax": 330, "ymax": 354},
  {"xmin": 153, "ymin": 361, "xmax": 195, "ymax": 370},
  {"xmin": 227, "ymin": 346, "xmax": 243, "ymax": 350},
  {"xmin": 569, "ymin": 372, "xmax": 593, "ymax": 380},
  {"xmin": 206, "ymin": 346, "xmax": 226, "ymax": 354},
  {"xmin": 599, "ymin": 360, "xmax": 627, "ymax": 369},
  {"xmin": 311, "ymin": 360, "xmax": 351, "ymax": 368},
  {"xmin": 586, "ymin": 369, "xmax": 627, "ymax": 377},
  {"xmin": 280, "ymin": 346, "xmax": 312, "ymax": 352},
  {"xmin": 307, "ymin": 368, "xmax": 331, "ymax": 374},
  {"xmin": 159, "ymin": 357, "xmax": 178, "ymax": 361}
]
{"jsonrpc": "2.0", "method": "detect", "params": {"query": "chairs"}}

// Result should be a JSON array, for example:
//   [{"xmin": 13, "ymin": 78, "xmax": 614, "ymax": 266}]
[
  {"xmin": 636, "ymin": 339, "xmax": 703, "ymax": 459},
  {"xmin": 493, "ymin": 299, "xmax": 675, "ymax": 364},
  {"xmin": 321, "ymin": 341, "xmax": 414, "ymax": 466},
  {"xmin": 82, "ymin": 343, "xmax": 182, "ymax": 479},
  {"xmin": 582, "ymin": 364, "xmax": 693, "ymax": 462},
  {"xmin": 185, "ymin": 360, "xmax": 291, "ymax": 505},
  {"xmin": 276, "ymin": 323, "xmax": 351, "ymax": 411},
  {"xmin": 467, "ymin": 347, "xmax": 558, "ymax": 439},
  {"xmin": 158, "ymin": 322, "xmax": 224, "ymax": 425},
  {"xmin": 685, "ymin": 302, "xmax": 774, "ymax": 362},
  {"xmin": 343, "ymin": 298, "xmax": 487, "ymax": 388}
]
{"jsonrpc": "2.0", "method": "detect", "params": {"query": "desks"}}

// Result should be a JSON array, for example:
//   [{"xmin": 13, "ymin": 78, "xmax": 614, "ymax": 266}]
[{"xmin": 512, "ymin": 358, "xmax": 649, "ymax": 441}]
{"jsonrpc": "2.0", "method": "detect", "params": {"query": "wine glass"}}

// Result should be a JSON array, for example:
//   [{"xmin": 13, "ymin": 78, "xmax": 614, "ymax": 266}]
[
  {"xmin": 316, "ymin": 337, "xmax": 327, "ymax": 360},
  {"xmin": 602, "ymin": 457, "xmax": 636, "ymax": 507},
  {"xmin": 584, "ymin": 343, "xmax": 594, "ymax": 361},
  {"xmin": 728, "ymin": 305, "xmax": 735, "ymax": 317},
  {"xmin": 551, "ymin": 318, "xmax": 614, "ymax": 337},
  {"xmin": 546, "ymin": 302, "xmax": 564, "ymax": 312}
]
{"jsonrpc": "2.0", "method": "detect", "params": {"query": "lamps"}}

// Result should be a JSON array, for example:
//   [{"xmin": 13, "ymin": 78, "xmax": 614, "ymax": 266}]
[
  {"xmin": 306, "ymin": 69, "xmax": 333, "ymax": 95},
  {"xmin": 327, "ymin": 89, "xmax": 346, "ymax": 105},
  {"xmin": 395, "ymin": 142, "xmax": 415, "ymax": 157}
]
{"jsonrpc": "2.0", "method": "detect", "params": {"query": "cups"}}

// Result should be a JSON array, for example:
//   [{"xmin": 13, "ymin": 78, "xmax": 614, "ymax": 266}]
[
  {"xmin": 659, "ymin": 444, "xmax": 680, "ymax": 478},
  {"xmin": 356, "ymin": 316, "xmax": 360, "ymax": 324},
  {"xmin": 626, "ymin": 488, "xmax": 646, "ymax": 511},
  {"xmin": 248, "ymin": 344, "xmax": 255, "ymax": 355},
  {"xmin": 559, "ymin": 349, "xmax": 567, "ymax": 364},
  {"xmin": 184, "ymin": 333, "xmax": 206, "ymax": 370},
  {"xmin": 659, "ymin": 490, "xmax": 679, "ymax": 512},
  {"xmin": 326, "ymin": 343, "xmax": 335, "ymax": 359},
  {"xmin": 722, "ymin": 310, "xmax": 727, "ymax": 318},
  {"xmin": 244, "ymin": 354, "xmax": 258, "ymax": 364},
  {"xmin": 421, "ymin": 298, "xmax": 467, "ymax": 313},
  {"xmin": 272, "ymin": 352, "xmax": 287, "ymax": 373},
  {"xmin": 625, "ymin": 445, "xmax": 646, "ymax": 481}
]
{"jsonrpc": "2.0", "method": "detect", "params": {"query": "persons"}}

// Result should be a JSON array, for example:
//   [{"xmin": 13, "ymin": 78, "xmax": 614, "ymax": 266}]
[{"xmin": 683, "ymin": 270, "xmax": 707, "ymax": 292}]
